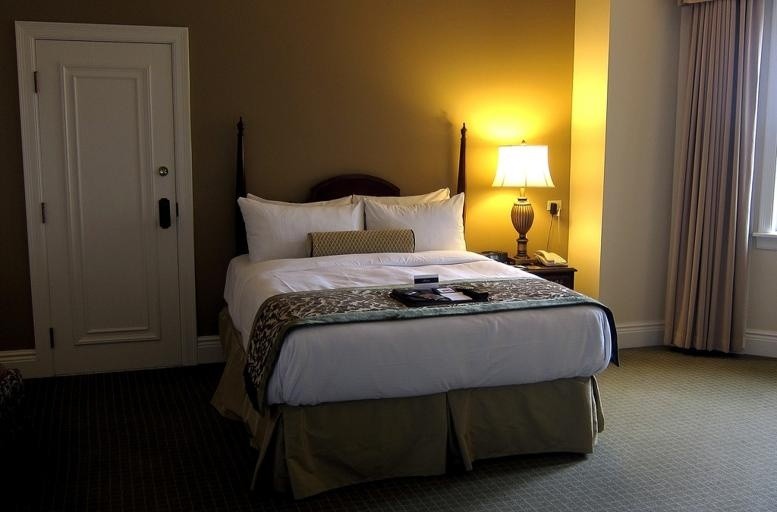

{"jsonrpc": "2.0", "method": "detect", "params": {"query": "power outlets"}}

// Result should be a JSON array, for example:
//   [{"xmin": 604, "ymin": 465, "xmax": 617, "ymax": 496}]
[{"xmin": 547, "ymin": 200, "xmax": 561, "ymax": 210}]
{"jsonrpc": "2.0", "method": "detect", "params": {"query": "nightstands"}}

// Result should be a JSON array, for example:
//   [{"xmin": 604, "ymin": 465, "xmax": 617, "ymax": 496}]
[{"xmin": 502, "ymin": 260, "xmax": 578, "ymax": 291}]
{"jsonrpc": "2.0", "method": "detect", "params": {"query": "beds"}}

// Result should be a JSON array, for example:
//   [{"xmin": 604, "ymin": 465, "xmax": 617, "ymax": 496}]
[{"xmin": 209, "ymin": 116, "xmax": 620, "ymax": 501}]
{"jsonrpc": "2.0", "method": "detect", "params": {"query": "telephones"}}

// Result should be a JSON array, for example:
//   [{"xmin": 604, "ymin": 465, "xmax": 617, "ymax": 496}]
[{"xmin": 533, "ymin": 250, "xmax": 569, "ymax": 267}]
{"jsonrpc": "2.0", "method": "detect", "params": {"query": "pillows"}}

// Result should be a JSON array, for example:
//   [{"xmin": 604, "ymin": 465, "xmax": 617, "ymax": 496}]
[
  {"xmin": 364, "ymin": 191, "xmax": 467, "ymax": 252},
  {"xmin": 236, "ymin": 196, "xmax": 365, "ymax": 263},
  {"xmin": 353, "ymin": 187, "xmax": 450, "ymax": 204},
  {"xmin": 307, "ymin": 229, "xmax": 416, "ymax": 257},
  {"xmin": 246, "ymin": 191, "xmax": 353, "ymax": 208}
]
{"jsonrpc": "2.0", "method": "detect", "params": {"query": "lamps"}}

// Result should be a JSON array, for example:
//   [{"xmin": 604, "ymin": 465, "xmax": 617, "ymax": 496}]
[{"xmin": 491, "ymin": 146, "xmax": 556, "ymax": 265}]
